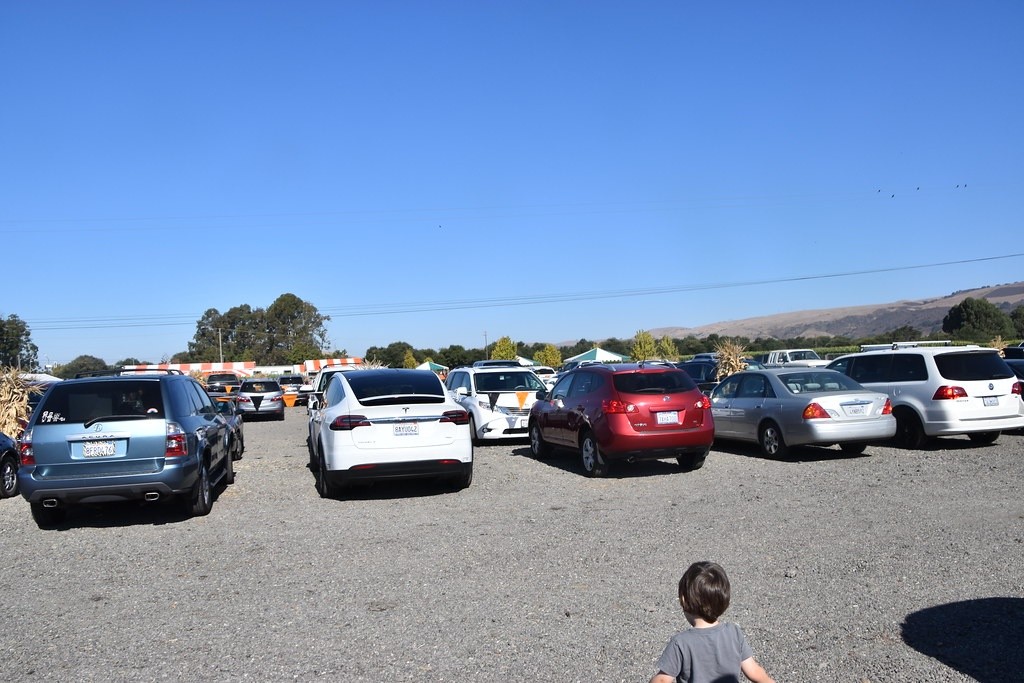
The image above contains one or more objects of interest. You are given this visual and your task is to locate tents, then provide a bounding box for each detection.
[417,361,450,378]
[563,346,632,364]
[511,354,541,367]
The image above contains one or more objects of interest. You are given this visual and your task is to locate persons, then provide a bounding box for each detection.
[649,561,775,683]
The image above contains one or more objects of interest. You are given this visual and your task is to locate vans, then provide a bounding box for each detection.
[443,365,552,446]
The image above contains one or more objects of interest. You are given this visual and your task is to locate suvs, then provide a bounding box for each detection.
[17,368,238,531]
[806,340,1024,451]
[526,361,716,479]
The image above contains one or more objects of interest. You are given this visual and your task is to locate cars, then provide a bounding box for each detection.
[205,341,860,461]
[0,431,21,499]
[706,366,898,462]
[308,367,475,498]
